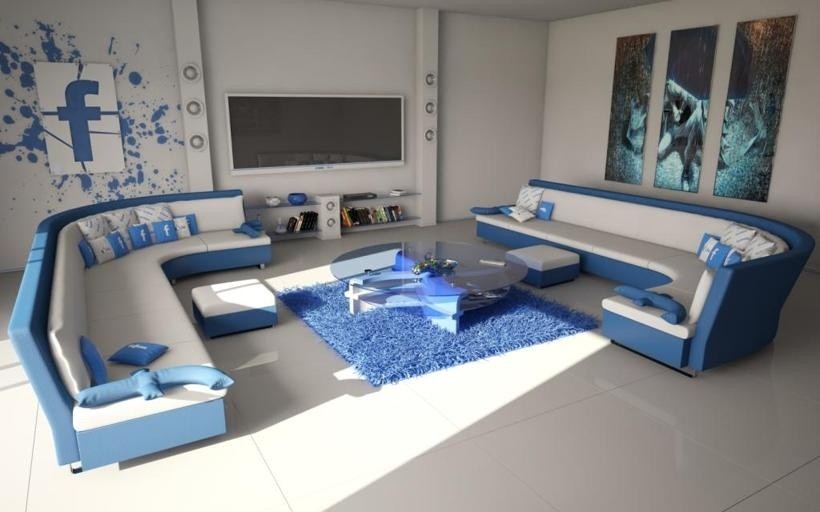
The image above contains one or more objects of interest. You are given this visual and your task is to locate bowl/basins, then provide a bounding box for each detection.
[287,192,308,206]
[264,196,281,207]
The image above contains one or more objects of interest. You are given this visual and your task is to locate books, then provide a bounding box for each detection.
[287,204,404,233]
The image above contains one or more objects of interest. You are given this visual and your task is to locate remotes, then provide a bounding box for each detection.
[479,258,507,267]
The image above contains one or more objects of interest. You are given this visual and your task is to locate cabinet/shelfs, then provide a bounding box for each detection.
[244,193,425,241]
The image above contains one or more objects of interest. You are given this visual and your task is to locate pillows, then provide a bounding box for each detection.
[231,219,263,239]
[77,333,237,407]
[695,222,777,271]
[471,186,555,224]
[76,202,202,268]
[613,283,687,325]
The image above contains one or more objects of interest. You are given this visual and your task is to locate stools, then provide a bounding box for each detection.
[504,245,582,290]
[191,278,278,339]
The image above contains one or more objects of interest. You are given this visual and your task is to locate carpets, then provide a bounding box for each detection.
[276,263,601,387]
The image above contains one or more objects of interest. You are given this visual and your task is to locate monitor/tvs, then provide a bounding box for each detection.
[224,94,405,176]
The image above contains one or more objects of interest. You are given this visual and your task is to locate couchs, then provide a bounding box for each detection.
[475,177,815,379]
[7,189,273,474]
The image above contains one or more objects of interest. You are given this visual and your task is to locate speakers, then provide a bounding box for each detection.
[424,71,437,144]
[180,63,208,152]
[316,195,341,241]
